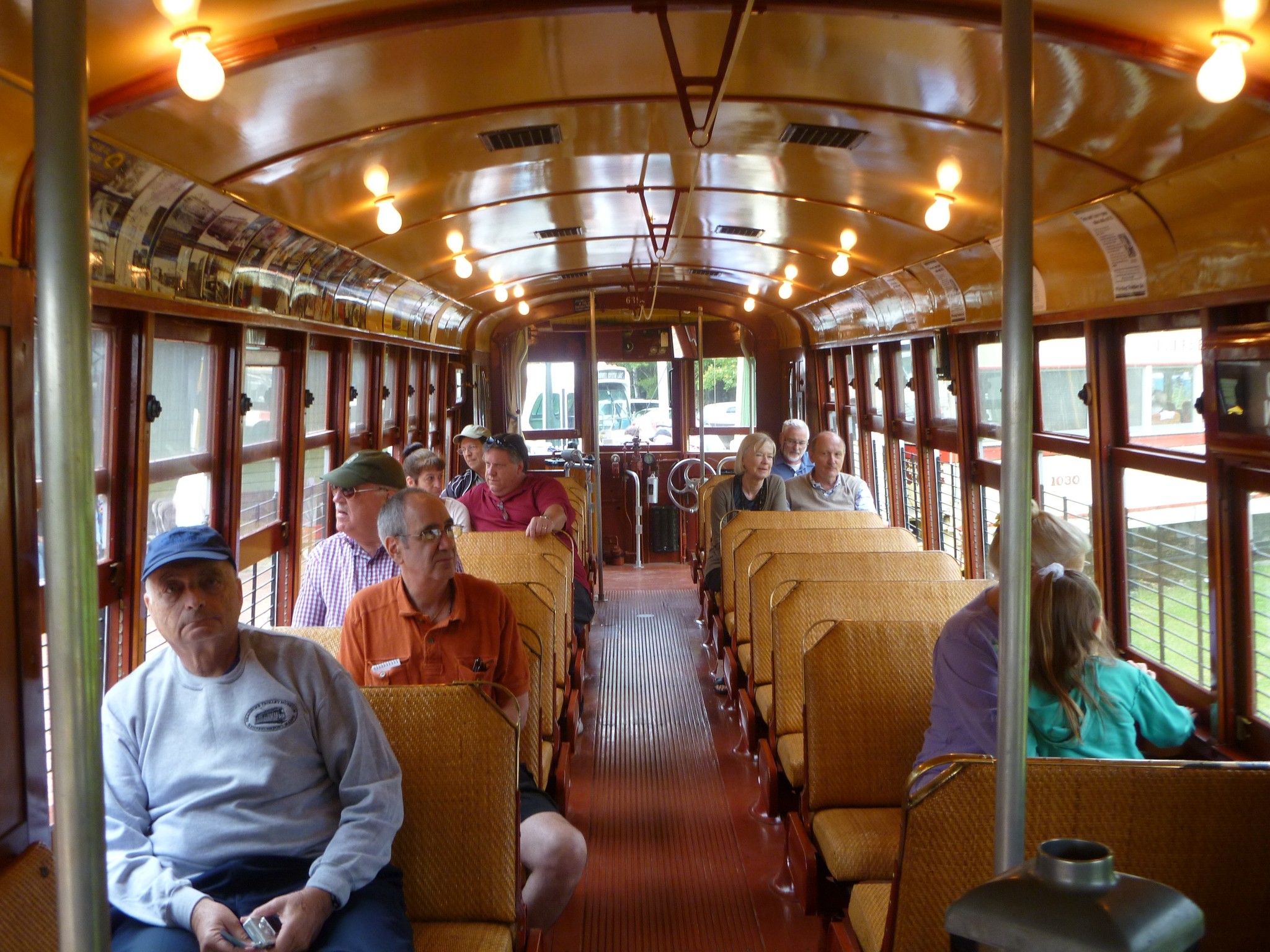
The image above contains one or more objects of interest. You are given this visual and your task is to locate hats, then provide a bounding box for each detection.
[141,525,236,582]
[318,450,407,489]
[453,424,491,444]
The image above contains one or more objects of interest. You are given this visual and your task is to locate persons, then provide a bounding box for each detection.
[440,424,493,500]
[338,489,588,931]
[455,433,595,640]
[103,526,415,952]
[290,448,465,628]
[907,498,1198,794]
[783,431,877,513]
[770,418,815,481]
[400,442,471,534]
[703,432,790,695]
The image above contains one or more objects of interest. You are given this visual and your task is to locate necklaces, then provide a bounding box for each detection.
[739,477,763,511]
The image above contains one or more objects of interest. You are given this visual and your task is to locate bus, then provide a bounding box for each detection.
[833,328,1270,531]
[519,361,631,456]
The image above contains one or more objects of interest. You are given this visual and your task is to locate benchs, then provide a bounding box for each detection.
[691,476,1270,952]
[262,477,597,952]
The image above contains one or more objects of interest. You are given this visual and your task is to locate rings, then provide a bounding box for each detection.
[542,526,547,529]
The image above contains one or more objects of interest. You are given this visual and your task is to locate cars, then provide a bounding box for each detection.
[598,398,738,451]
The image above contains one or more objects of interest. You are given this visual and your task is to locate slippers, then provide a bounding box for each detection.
[714,677,728,694]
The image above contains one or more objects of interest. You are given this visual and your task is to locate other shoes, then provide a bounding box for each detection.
[577,717,583,734]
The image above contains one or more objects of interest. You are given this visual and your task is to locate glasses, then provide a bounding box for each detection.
[457,443,485,455]
[783,434,808,448]
[393,524,464,542]
[496,498,509,520]
[330,483,389,499]
[486,436,522,455]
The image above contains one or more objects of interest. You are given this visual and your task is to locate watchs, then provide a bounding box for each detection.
[540,515,549,519]
